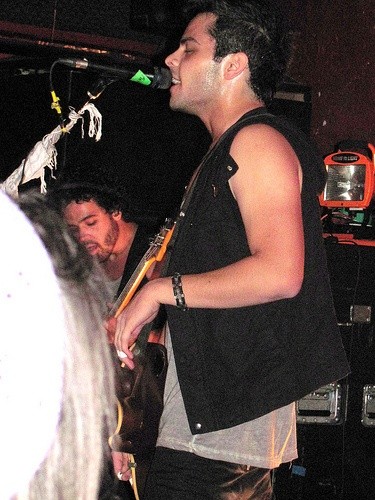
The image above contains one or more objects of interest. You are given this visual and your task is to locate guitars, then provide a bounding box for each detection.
[104,217,177,323]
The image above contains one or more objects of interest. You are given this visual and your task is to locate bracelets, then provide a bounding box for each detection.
[170,269,189,313]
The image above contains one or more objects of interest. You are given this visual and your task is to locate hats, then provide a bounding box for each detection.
[52,164,121,194]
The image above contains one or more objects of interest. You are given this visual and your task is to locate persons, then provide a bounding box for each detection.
[113,0,349,499]
[1,187,115,500]
[51,165,168,343]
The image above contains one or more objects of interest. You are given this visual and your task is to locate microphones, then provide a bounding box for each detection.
[56,57,172,90]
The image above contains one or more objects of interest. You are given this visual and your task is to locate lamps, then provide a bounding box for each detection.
[318,139,375,240]
